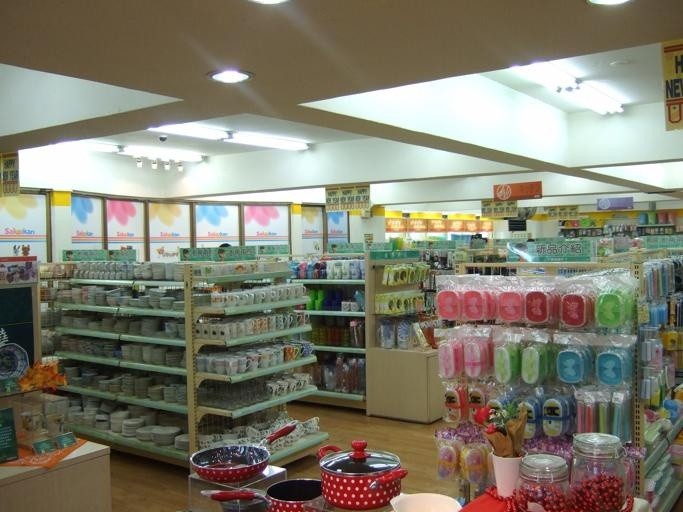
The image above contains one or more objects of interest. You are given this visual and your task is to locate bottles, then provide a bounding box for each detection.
[334,301,365,394]
[642,259,676,326]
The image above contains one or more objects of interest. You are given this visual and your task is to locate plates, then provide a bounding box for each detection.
[390,492,463,512]
[122,417,189,452]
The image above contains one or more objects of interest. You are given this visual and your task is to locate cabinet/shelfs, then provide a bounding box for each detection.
[1,433,111,512]
[41,248,682,512]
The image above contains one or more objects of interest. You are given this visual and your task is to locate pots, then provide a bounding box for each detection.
[189,422,407,512]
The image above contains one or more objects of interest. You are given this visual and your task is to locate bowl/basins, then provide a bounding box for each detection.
[62,285,186,406]
[110,411,130,434]
[0,343,30,381]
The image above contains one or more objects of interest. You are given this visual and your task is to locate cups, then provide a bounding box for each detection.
[73,260,184,282]
[492,451,527,499]
[21,411,64,436]
[374,319,418,351]
[197,282,321,456]
